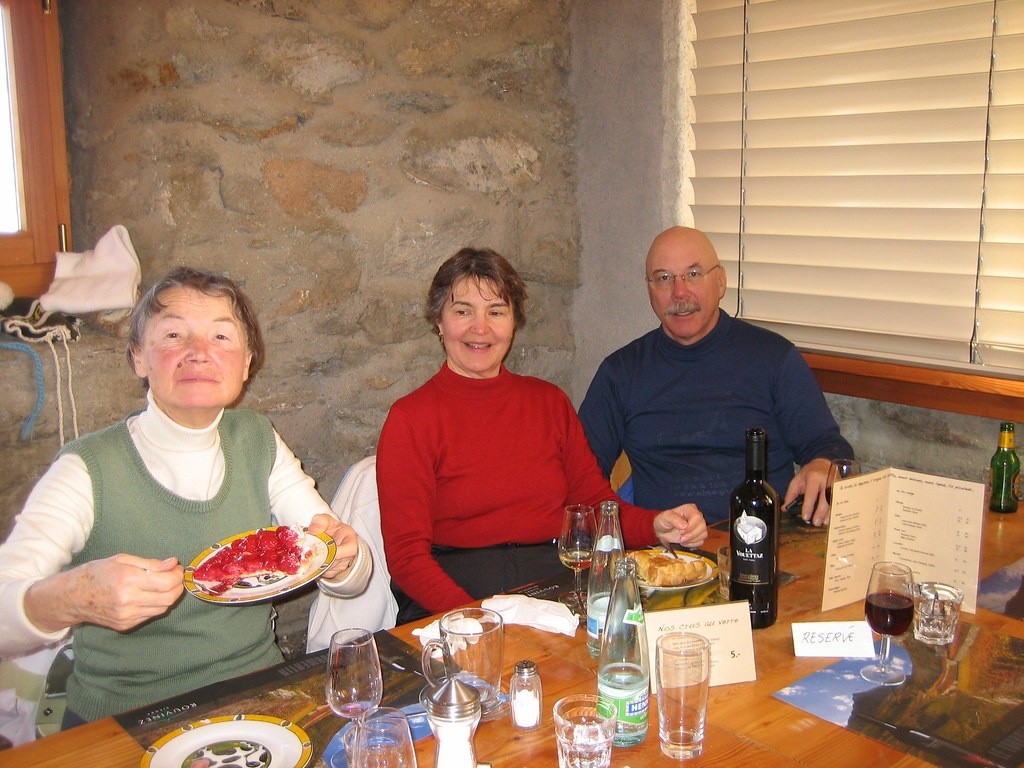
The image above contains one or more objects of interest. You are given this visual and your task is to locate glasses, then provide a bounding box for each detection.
[645,265,724,291]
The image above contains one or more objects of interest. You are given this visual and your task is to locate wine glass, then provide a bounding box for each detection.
[859,562,914,685]
[325,627,383,767]
[557,504,597,608]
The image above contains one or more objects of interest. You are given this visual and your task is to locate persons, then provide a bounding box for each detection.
[376,247,709,626]
[0,268,374,737]
[577,226,854,525]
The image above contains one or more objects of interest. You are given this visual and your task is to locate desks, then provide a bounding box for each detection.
[0,489,1024,768]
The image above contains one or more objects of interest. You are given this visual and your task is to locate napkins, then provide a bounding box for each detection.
[481,593,580,638]
[412,611,483,658]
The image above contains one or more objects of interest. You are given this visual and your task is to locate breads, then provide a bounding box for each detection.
[628,549,708,585]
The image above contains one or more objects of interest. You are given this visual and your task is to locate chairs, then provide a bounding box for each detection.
[301,454,399,654]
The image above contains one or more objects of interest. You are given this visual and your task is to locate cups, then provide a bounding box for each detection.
[716,546,730,600]
[824,459,863,508]
[654,632,711,759]
[553,694,617,768]
[913,581,964,645]
[343,707,418,768]
[420,608,503,704]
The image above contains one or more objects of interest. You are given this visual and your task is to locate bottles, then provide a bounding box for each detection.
[585,502,625,660]
[426,678,482,768]
[989,422,1019,515]
[728,427,779,629]
[597,557,649,746]
[509,660,542,729]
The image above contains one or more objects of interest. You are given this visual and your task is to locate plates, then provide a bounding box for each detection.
[182,527,337,603]
[627,549,719,591]
[418,673,511,721]
[139,713,314,768]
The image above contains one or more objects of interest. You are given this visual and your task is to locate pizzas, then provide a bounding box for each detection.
[192,524,310,585]
